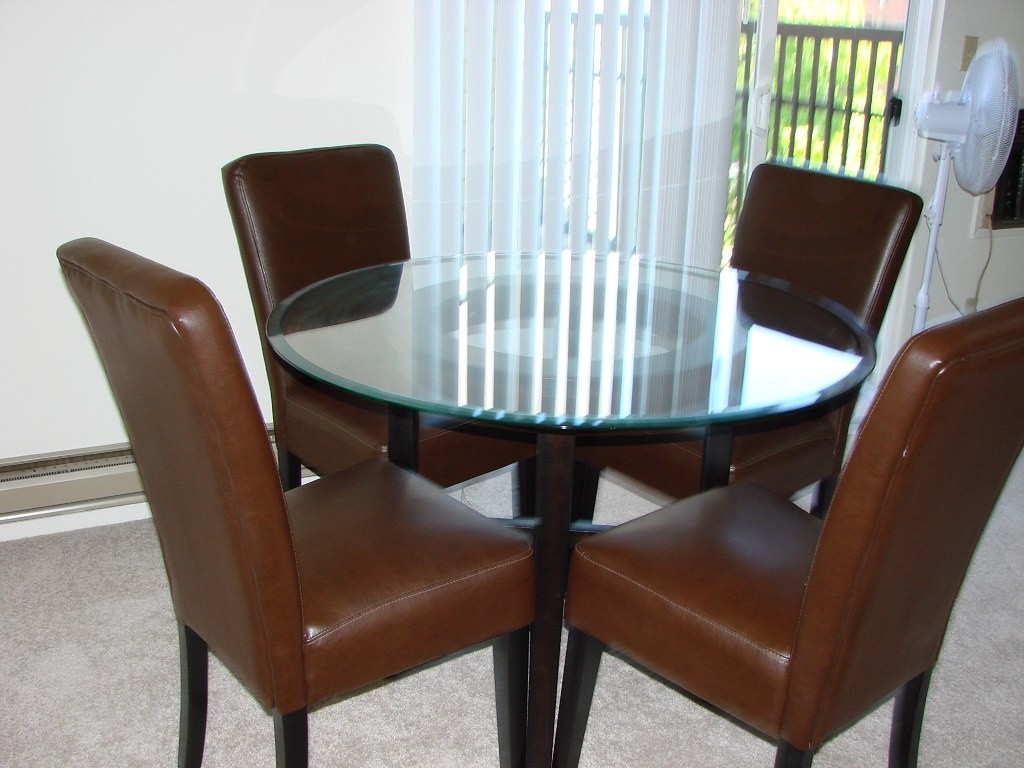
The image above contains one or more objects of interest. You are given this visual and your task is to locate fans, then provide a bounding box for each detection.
[833,38,1020,434]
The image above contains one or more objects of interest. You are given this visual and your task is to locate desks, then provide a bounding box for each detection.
[267,254,879,768]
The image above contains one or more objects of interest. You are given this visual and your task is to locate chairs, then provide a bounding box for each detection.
[57,144,1024,768]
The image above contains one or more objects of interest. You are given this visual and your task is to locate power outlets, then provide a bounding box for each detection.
[965,298,977,315]
